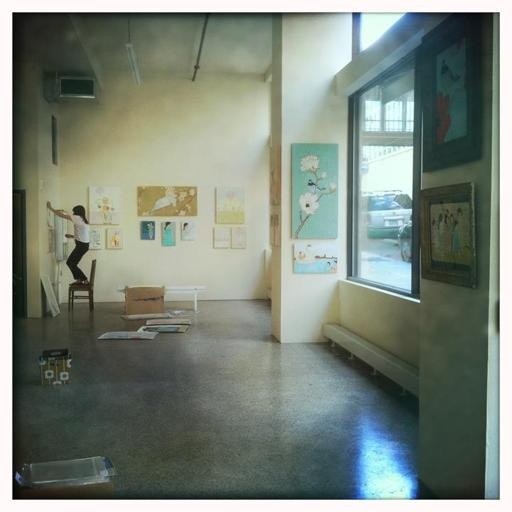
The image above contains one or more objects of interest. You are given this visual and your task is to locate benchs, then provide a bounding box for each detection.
[117,285,209,312]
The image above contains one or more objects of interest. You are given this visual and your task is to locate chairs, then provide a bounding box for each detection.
[68,259,97,312]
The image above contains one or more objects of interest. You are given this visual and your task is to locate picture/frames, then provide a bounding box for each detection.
[416,13,482,291]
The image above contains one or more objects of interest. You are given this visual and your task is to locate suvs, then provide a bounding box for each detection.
[358,188,415,241]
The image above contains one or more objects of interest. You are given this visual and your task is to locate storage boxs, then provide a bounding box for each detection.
[123,284,165,315]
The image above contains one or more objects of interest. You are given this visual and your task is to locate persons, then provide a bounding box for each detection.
[47,201,90,286]
[431,208,471,258]
